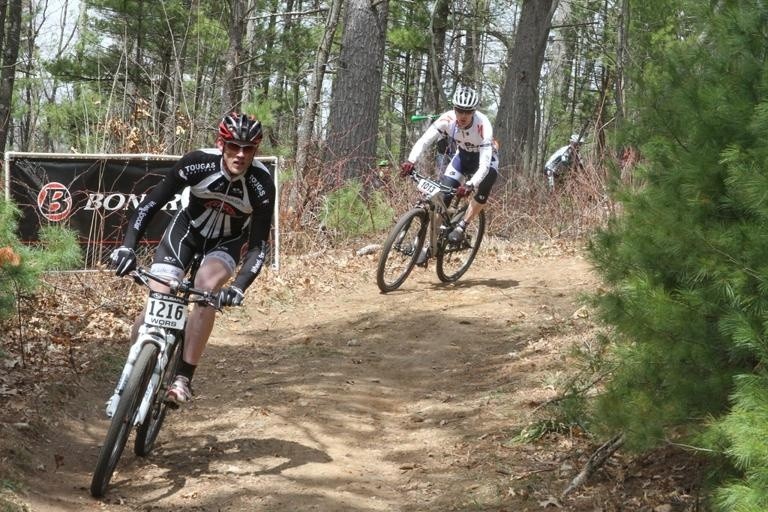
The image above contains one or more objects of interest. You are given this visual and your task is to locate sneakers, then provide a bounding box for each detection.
[414,249,433,265]
[447,225,467,244]
[162,375,195,405]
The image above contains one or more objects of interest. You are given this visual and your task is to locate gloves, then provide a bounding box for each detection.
[401,160,415,176]
[110,246,136,277]
[453,183,475,199]
[213,285,243,312]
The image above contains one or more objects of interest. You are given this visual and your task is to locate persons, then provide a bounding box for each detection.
[397,84,501,267]
[539,131,584,196]
[102,109,277,420]
[435,135,451,170]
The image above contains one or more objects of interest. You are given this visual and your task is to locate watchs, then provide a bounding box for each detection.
[464,180,475,189]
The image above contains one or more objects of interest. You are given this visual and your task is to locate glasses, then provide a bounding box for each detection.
[454,108,475,115]
[224,142,257,156]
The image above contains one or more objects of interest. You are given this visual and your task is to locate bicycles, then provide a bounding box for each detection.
[89,250,245,500]
[376,166,486,292]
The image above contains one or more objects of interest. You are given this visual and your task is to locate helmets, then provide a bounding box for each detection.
[569,133,585,144]
[452,86,479,110]
[218,111,264,144]
